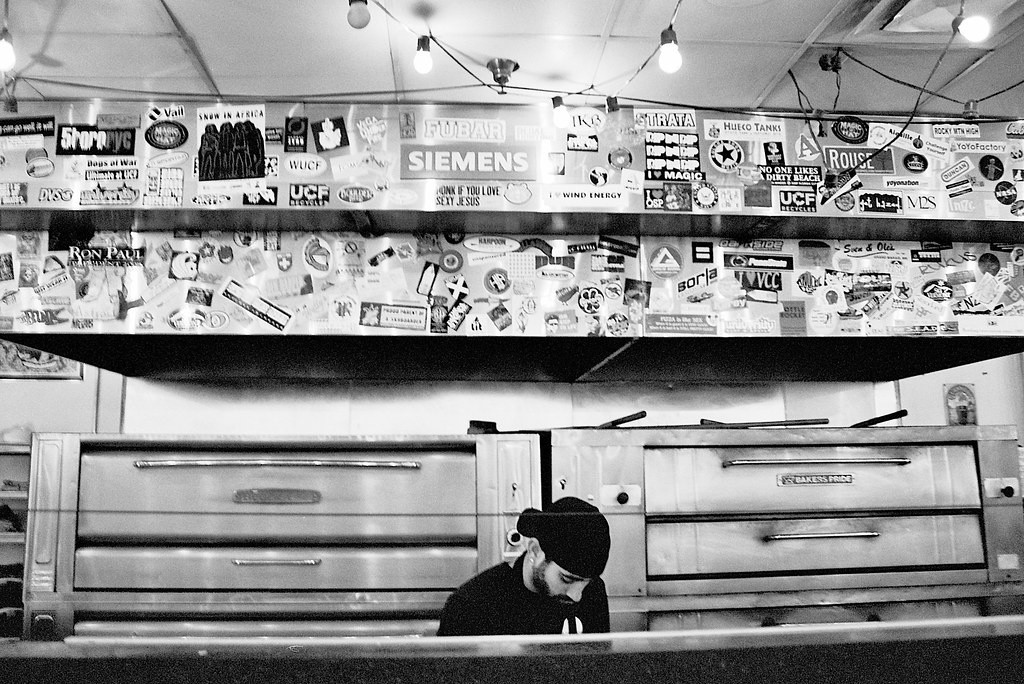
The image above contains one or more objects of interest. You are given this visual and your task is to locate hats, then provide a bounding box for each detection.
[516,497,611,577]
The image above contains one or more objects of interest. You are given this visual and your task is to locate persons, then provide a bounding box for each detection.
[432,498,611,633]
